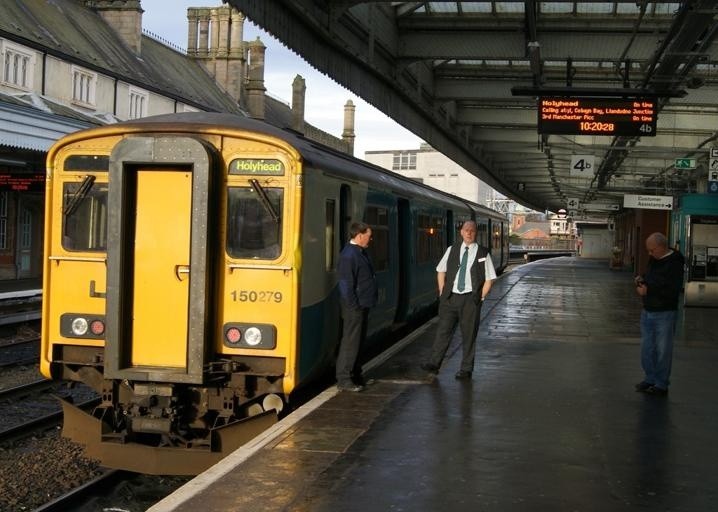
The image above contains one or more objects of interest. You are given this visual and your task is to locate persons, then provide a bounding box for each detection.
[633,230,683,398]
[421,219,497,382]
[332,220,379,393]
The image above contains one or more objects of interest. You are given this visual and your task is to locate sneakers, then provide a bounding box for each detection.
[418,362,439,375]
[454,371,472,378]
[634,381,670,402]
[338,375,375,392]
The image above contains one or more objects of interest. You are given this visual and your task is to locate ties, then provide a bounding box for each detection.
[456,245,471,292]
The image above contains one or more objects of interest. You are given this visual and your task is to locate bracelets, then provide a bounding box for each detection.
[480,297,486,301]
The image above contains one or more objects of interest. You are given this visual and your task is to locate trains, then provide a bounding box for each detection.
[35,110,514,479]
